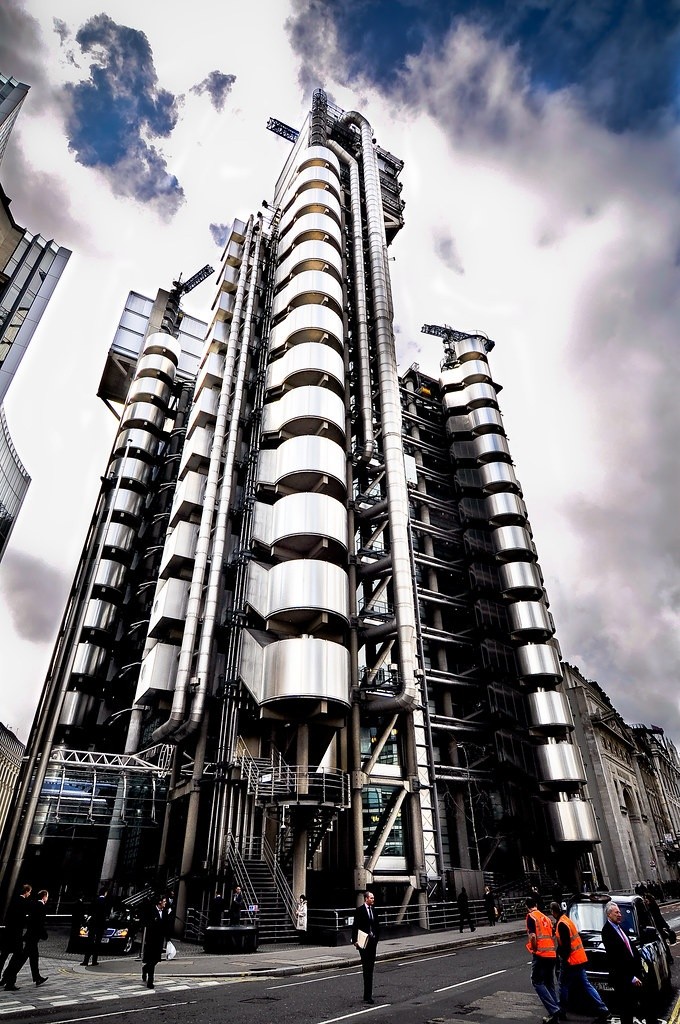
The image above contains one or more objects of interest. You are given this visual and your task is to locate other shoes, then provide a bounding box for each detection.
[36,977,49,986]
[460,930,463,933]
[543,1013,560,1024]
[4,985,20,991]
[142,967,147,981]
[80,963,88,966]
[92,963,99,966]
[147,984,154,989]
[670,958,673,965]
[592,1011,613,1024]
[363,996,374,1004]
[472,928,477,932]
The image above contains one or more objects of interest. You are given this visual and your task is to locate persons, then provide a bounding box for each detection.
[0,884,49,991]
[581,881,590,892]
[484,887,504,927]
[635,879,680,902]
[643,893,676,964]
[458,887,476,933]
[552,882,563,906]
[207,891,225,926]
[142,891,177,989]
[532,887,546,910]
[229,886,243,926]
[350,891,379,1003]
[593,880,609,891]
[601,902,658,1024]
[549,902,612,1024]
[296,894,307,945]
[526,897,564,1024]
[71,887,108,966]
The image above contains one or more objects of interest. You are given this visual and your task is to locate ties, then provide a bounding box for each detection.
[368,907,374,936]
[618,926,631,951]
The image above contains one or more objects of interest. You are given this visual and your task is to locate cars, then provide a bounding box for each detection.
[561,893,673,1012]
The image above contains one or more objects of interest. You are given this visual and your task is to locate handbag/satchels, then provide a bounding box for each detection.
[165,941,176,960]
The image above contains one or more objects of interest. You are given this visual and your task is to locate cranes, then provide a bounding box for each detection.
[266,117,302,144]
[171,264,215,299]
[421,323,497,370]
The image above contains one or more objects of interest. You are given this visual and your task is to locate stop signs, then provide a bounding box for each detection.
[649,861,655,867]
